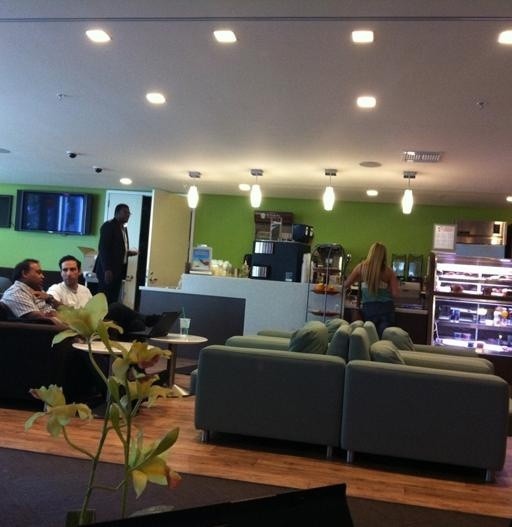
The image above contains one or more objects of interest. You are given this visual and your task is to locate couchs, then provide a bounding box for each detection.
[0,277,144,417]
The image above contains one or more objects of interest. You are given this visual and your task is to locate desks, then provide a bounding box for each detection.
[71,340,162,419]
[145,328,213,401]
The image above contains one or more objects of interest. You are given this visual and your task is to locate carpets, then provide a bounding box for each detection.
[0,446,512,527]
[173,363,199,378]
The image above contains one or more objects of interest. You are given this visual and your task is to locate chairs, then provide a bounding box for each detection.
[193,315,346,469]
[349,315,497,376]
[73,480,356,527]
[342,322,510,489]
[256,315,345,338]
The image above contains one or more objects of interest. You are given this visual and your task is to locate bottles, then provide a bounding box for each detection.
[493,307,511,324]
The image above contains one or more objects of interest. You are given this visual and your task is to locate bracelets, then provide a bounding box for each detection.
[46,294,54,304]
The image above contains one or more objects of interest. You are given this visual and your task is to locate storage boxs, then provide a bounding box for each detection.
[77,246,98,271]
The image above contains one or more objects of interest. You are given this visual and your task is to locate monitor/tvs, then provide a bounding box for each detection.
[14,190,93,235]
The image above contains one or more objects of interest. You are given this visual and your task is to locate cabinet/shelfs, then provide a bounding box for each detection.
[424,247,512,379]
[304,241,348,326]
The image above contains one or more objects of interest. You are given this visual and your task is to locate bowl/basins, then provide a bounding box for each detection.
[291,221,315,242]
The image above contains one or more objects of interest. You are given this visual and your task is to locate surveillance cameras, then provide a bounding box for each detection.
[67,152,77,160]
[93,166,104,175]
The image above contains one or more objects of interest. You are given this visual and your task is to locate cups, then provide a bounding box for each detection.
[463,332,471,340]
[210,257,240,276]
[285,271,293,281]
[179,316,191,337]
[455,332,462,340]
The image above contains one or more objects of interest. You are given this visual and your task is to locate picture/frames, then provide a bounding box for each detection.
[432,221,459,252]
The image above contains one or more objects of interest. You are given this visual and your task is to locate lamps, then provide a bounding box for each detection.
[246,168,265,208]
[397,169,417,216]
[185,170,203,209]
[320,167,338,211]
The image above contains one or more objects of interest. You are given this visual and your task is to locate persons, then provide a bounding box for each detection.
[0,259,66,320]
[46,255,93,309]
[92,204,137,302]
[345,242,399,340]
